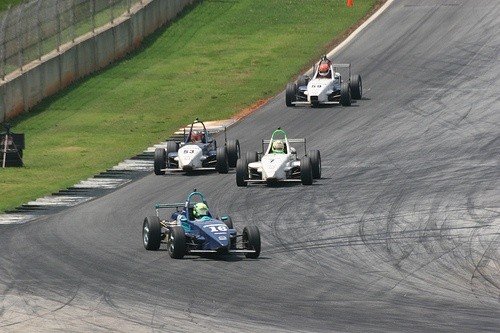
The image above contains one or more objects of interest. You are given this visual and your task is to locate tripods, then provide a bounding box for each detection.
[0,133,25,169]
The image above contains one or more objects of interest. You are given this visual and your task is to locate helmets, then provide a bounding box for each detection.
[272,141,284,153]
[193,203,209,217]
[319,64,331,77]
[191,131,200,140]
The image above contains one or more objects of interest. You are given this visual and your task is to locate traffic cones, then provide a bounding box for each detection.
[347,0,353,7]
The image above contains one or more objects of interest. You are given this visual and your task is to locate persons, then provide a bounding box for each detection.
[319,64,330,78]
[193,202,208,220]
[272,140,284,154]
[191,131,200,143]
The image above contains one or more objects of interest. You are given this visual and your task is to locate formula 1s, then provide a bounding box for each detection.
[286,53,362,107]
[142,188,261,259]
[235,127,322,187]
[153,118,241,175]
